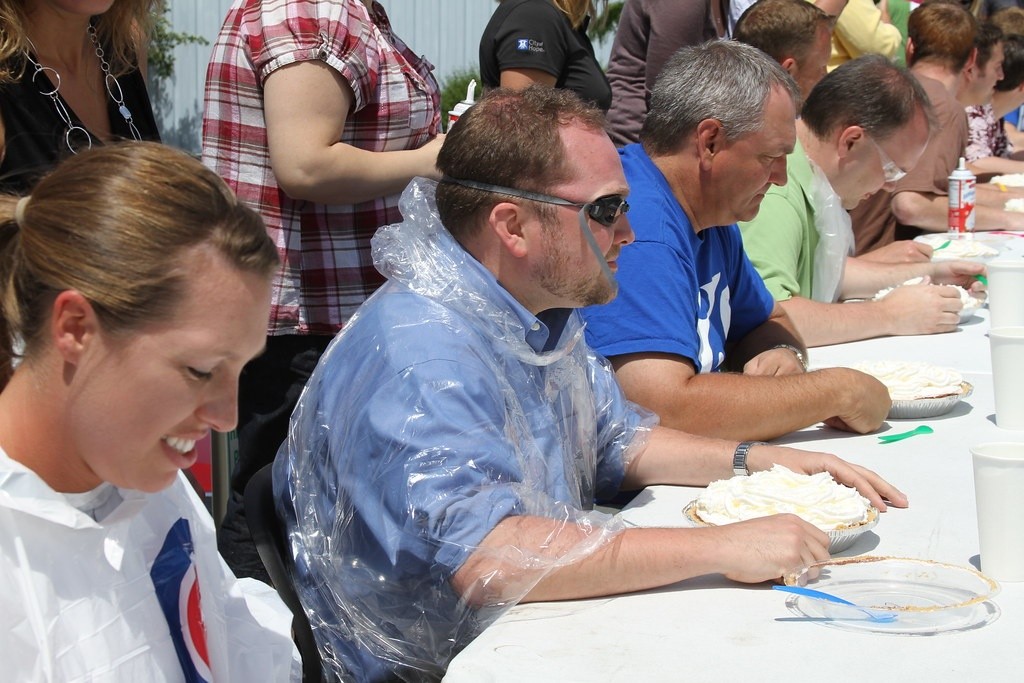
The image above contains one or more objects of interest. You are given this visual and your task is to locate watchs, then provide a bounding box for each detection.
[734,440,767,476]
[769,343,808,370]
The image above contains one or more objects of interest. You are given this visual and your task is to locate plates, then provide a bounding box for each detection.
[806,356,972,417]
[682,497,880,556]
[958,301,981,325]
[783,556,996,634]
[913,233,1024,264]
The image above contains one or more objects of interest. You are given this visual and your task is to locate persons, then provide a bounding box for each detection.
[604,0,1024,258]
[473,0,617,121]
[202,0,451,586]
[0,143,304,683]
[734,50,988,349]
[272,81,908,683]
[0,0,205,397]
[573,35,894,443]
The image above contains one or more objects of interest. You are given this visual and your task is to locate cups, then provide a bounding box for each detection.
[983,259,1024,332]
[969,439,1024,582]
[986,326,1024,430]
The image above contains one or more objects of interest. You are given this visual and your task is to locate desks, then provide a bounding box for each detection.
[450,168,1024,681]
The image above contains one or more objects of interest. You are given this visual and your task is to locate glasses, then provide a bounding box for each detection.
[589,193,632,227]
[863,130,908,183]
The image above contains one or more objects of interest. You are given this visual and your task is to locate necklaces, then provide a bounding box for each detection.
[15,17,142,155]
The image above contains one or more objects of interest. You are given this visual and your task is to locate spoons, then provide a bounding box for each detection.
[772,584,897,624]
[877,425,933,443]
[932,239,951,251]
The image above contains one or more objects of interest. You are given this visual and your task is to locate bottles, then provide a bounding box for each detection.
[946,157,975,233]
[447,79,478,136]
